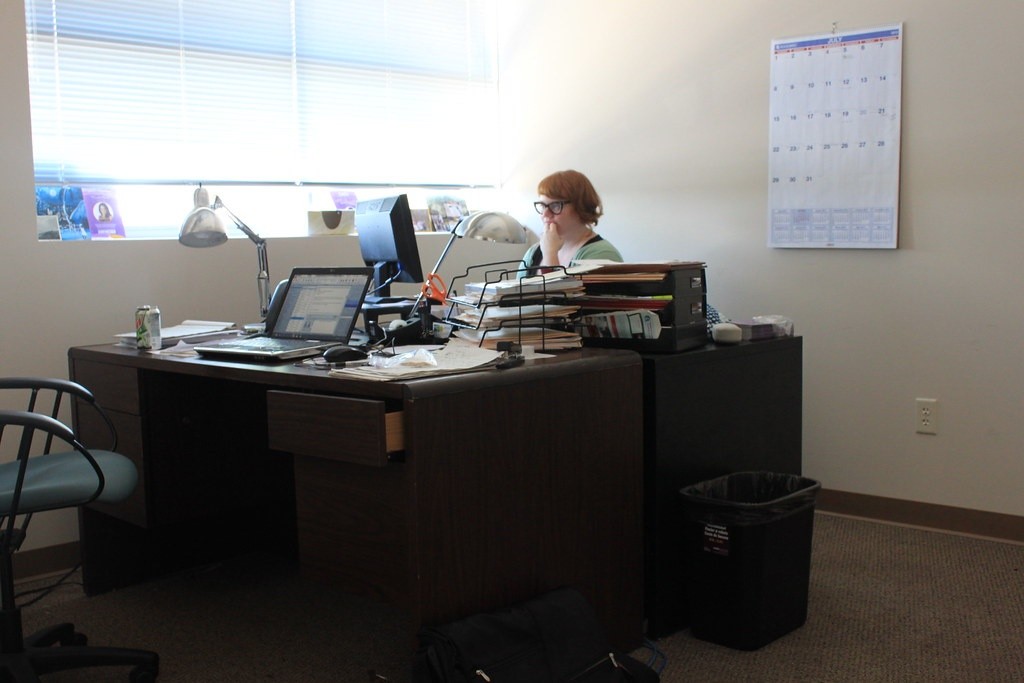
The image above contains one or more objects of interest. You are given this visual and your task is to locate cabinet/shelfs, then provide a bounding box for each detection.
[574,259,707,356]
[445,260,583,355]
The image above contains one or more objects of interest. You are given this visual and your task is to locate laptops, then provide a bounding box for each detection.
[193,267,375,361]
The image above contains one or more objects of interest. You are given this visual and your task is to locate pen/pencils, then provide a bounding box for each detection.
[314,361,370,371]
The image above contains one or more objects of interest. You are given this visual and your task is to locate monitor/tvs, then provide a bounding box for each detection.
[355,194,424,304]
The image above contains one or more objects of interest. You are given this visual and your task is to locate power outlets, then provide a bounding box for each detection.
[915,398,938,435]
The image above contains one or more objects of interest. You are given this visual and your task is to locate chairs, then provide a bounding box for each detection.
[0,376,163,683]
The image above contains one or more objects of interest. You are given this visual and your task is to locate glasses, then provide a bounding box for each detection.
[534,200,573,214]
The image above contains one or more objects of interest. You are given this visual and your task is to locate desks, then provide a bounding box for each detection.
[68,321,803,683]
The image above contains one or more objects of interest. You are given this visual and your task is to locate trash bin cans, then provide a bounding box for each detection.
[680,472,822,652]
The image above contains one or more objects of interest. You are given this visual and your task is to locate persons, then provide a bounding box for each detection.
[517,170,624,277]
[98,204,112,222]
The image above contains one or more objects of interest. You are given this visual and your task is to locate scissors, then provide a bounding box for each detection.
[422,272,448,305]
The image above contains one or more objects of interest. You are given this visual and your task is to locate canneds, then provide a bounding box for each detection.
[135,304,162,351]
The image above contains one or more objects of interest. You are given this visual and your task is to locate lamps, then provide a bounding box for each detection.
[408,211,527,318]
[178,187,273,323]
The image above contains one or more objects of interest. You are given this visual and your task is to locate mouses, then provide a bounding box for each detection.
[323,346,369,363]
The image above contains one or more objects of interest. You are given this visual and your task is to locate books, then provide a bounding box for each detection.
[444,278,586,349]
[112,319,237,350]
